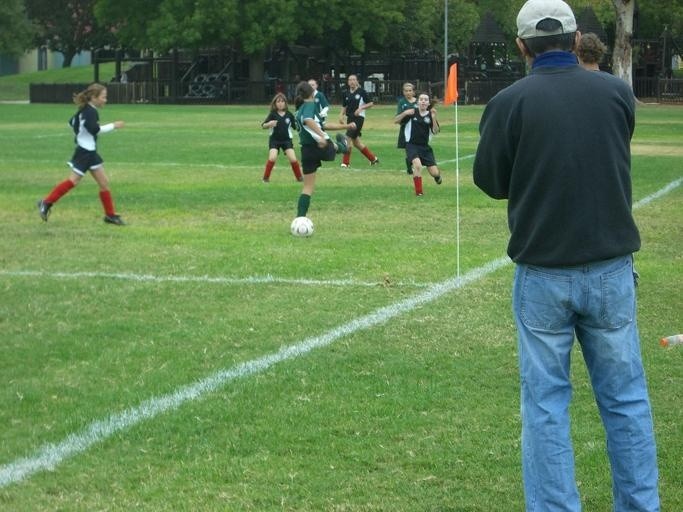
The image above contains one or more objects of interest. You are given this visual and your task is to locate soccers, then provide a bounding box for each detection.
[289,216,313,236]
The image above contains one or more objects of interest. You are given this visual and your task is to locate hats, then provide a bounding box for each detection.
[515,0,578,40]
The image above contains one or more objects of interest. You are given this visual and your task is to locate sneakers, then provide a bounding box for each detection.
[435,176,443,185]
[37,200,52,221]
[341,163,350,168]
[104,213,123,225]
[262,176,269,183]
[336,133,349,154]
[416,193,424,197]
[371,156,379,166]
[296,176,304,182]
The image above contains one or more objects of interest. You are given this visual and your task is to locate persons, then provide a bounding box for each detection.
[339,74,380,168]
[576,32,606,72]
[307,79,328,132]
[295,81,357,217]
[397,81,418,148]
[261,92,303,182]
[394,91,442,196]
[472,0,661,511]
[38,82,127,226]
